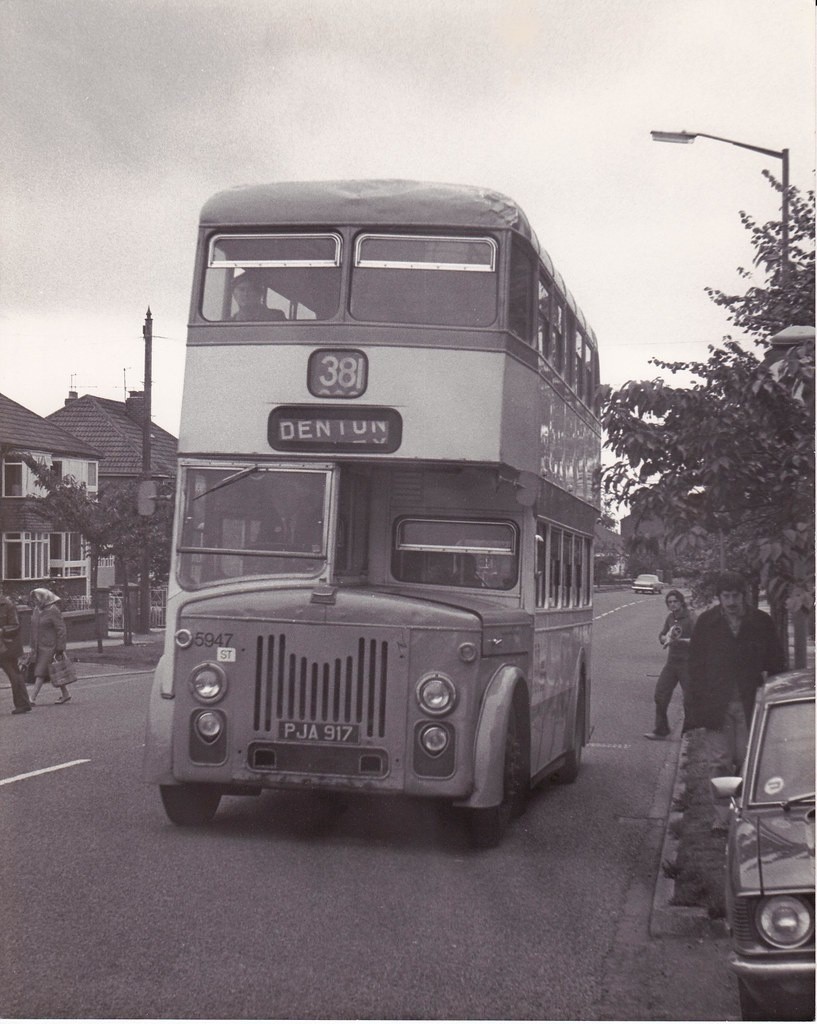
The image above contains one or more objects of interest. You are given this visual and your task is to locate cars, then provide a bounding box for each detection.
[710,664,817,1020]
[631,574,665,595]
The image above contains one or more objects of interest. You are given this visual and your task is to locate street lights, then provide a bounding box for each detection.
[649,127,805,663]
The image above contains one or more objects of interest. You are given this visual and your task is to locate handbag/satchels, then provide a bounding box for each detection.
[48,652,78,687]
[20,663,50,683]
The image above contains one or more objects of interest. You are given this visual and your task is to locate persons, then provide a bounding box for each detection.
[227,271,286,322]
[1,580,32,715]
[27,587,71,707]
[445,553,483,589]
[687,571,786,797]
[242,478,321,555]
[643,591,699,740]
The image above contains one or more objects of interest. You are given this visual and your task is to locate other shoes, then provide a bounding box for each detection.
[11,703,32,714]
[711,819,729,838]
[643,732,673,741]
[28,698,36,706]
[55,693,72,704]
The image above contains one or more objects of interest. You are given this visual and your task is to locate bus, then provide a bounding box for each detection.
[131,181,607,842]
